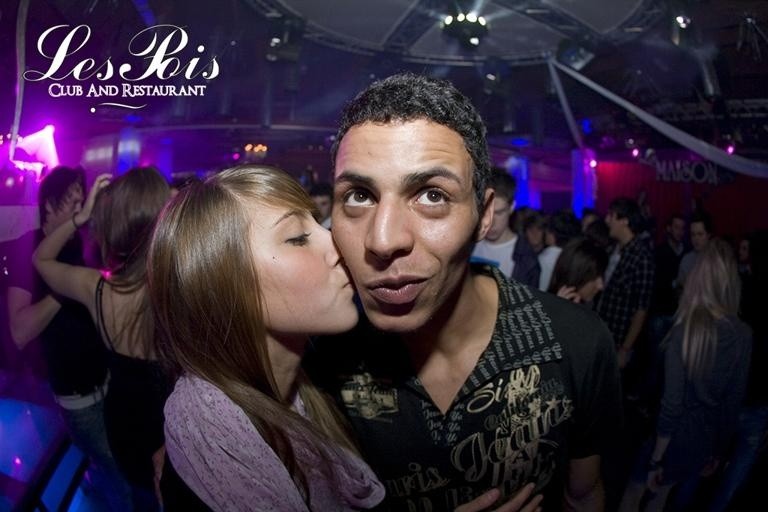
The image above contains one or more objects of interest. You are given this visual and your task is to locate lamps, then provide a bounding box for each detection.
[437,14,488,49]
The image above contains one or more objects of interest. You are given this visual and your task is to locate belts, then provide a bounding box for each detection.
[52,382,108,411]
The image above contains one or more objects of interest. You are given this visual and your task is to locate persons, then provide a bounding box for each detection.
[296,69,633,509]
[0,159,767,512]
